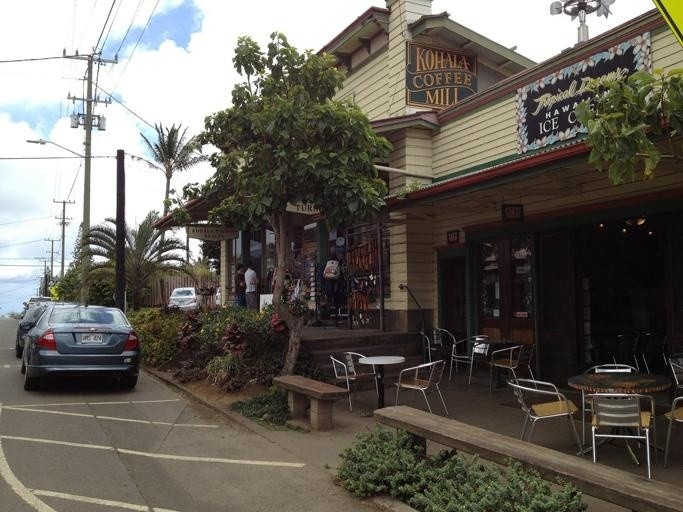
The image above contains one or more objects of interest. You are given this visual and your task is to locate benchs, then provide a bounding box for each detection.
[273,373,349,436]
[372,403,682,512]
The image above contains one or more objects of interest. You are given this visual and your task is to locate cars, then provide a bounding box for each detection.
[15,297,74,356]
[166,286,202,313]
[19,304,140,392]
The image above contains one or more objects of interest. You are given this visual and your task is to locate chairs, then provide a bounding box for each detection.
[489,344,539,392]
[506,378,587,460]
[584,393,656,480]
[420,327,458,374]
[611,326,682,397]
[393,358,450,418]
[583,363,638,375]
[663,396,682,466]
[449,335,491,384]
[329,351,381,411]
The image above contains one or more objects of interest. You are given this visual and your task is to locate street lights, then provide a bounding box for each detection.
[26,137,91,306]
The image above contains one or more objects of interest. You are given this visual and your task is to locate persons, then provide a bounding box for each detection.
[245,259,260,310]
[234,263,246,307]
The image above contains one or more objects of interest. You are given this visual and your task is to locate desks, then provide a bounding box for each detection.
[568,373,673,465]
[357,356,404,409]
